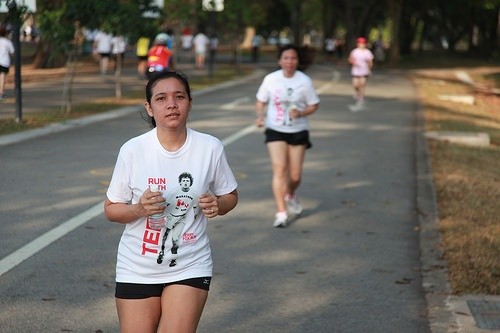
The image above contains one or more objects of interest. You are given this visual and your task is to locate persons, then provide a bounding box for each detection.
[134,29,177,87]
[180,26,219,70]
[84,24,126,78]
[251,33,261,63]
[104,70,240,333]
[324,33,347,62]
[0,24,15,98]
[347,37,374,106]
[256,43,320,227]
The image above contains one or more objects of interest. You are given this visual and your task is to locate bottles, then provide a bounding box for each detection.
[149,184,166,229]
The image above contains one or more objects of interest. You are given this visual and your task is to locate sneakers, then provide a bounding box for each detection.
[273,211,287,227]
[285,195,302,214]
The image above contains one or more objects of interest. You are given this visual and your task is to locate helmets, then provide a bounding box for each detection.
[155,34,168,44]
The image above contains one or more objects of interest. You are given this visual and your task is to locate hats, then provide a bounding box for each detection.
[357,37,366,43]
